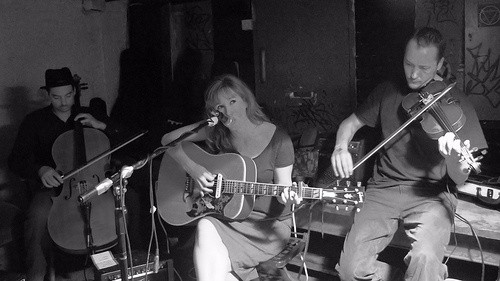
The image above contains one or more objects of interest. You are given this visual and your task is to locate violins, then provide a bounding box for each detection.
[403,80,487,175]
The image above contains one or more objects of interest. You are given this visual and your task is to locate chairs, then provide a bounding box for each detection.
[256,150,320,281]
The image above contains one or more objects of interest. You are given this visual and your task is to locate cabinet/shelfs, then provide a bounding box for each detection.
[291,190,500,280]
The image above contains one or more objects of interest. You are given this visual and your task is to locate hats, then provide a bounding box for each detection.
[41,66,79,90]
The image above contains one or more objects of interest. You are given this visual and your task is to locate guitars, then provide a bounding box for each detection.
[156,142,366,225]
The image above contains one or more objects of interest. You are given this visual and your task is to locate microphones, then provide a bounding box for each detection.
[210,110,234,126]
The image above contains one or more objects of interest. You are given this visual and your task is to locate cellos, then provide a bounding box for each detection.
[48,71,127,254]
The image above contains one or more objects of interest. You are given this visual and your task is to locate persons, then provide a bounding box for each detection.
[160,75,294,281]
[10,66,124,281]
[331,26,486,281]
